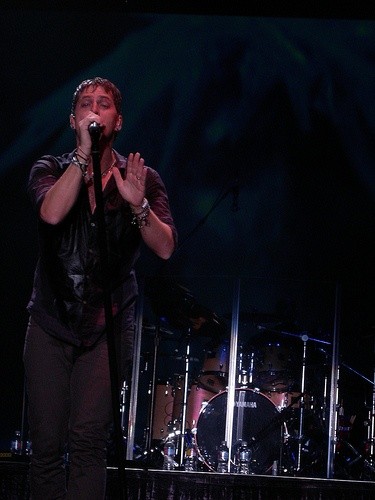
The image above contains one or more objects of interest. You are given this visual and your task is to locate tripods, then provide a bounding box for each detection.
[132,325,375,481]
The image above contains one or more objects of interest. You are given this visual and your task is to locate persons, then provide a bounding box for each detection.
[22,77,178,500]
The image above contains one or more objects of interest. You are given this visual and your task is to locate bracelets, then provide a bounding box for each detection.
[70,148,89,168]
[130,198,150,228]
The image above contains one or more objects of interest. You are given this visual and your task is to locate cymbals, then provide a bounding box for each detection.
[154,275,233,333]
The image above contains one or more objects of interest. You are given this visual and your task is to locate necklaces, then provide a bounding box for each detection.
[98,159,117,176]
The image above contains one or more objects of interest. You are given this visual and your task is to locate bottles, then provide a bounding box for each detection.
[24,431,32,455]
[161,436,176,471]
[184,438,198,472]
[237,441,250,474]
[12,431,22,455]
[217,440,228,473]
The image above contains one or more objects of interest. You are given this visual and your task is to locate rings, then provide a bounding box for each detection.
[137,177,141,180]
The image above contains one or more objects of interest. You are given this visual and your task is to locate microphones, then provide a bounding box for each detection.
[230,168,241,211]
[187,325,193,336]
[316,345,342,362]
[88,121,100,134]
[257,325,288,336]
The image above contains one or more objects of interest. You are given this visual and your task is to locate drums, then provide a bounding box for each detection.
[268,380,325,419]
[168,370,231,441]
[143,379,186,447]
[192,384,284,473]
